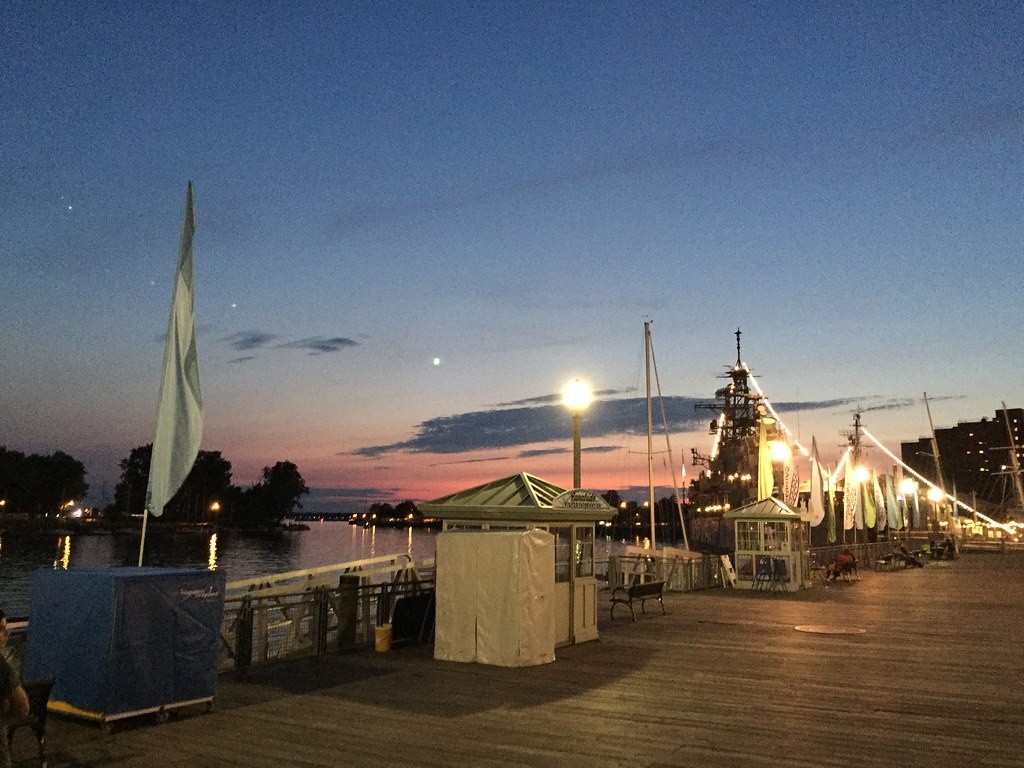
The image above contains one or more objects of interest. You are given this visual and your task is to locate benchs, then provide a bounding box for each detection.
[7,677,59,768]
[609,581,666,622]
[875,544,945,571]
[831,560,861,584]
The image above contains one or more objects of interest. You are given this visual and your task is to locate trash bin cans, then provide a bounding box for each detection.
[375,624,392,653]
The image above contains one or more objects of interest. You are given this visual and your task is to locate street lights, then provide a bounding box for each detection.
[560,378,594,576]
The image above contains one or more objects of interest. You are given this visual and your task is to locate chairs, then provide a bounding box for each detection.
[751,556,790,596]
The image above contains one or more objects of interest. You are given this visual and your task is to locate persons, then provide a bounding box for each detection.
[825,549,855,580]
[893,538,953,568]
[0,610,30,768]
